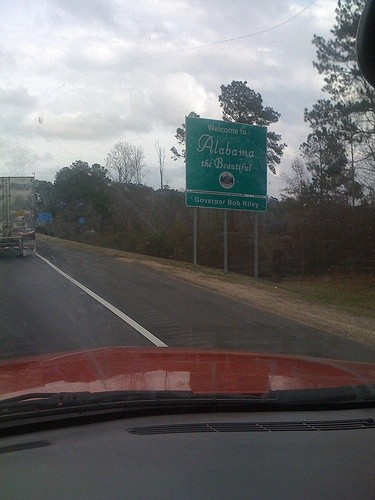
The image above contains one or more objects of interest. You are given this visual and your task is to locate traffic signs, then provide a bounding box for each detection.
[184,116,268,197]
[184,191,267,213]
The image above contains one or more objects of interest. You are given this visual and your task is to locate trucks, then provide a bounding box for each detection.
[0,175,37,258]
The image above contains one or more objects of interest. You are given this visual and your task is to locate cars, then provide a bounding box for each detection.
[0,347,375,500]
